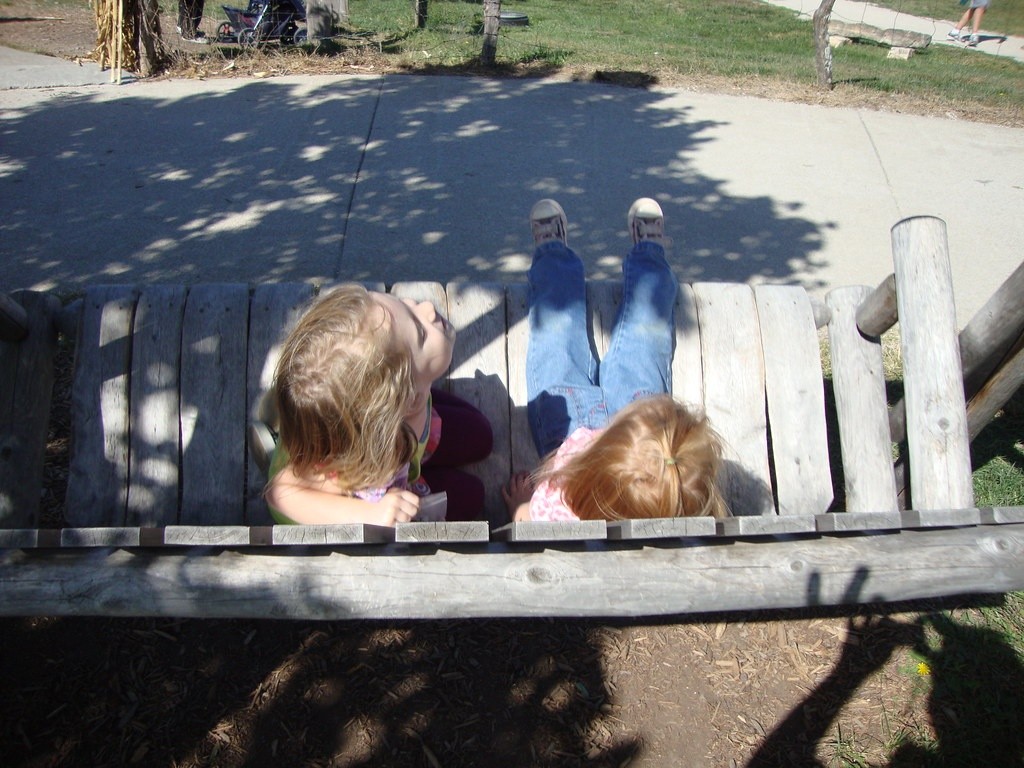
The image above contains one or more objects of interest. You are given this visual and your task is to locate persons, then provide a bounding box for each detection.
[944,0,991,47]
[499,199,733,524]
[243,282,493,529]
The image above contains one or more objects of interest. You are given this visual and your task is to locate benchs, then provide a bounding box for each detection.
[0,214,1024,621]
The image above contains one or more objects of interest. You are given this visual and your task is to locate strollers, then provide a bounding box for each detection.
[215,0,308,50]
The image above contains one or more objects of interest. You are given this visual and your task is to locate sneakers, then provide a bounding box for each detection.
[628,198,674,249]
[964,33,979,46]
[947,31,963,42]
[529,199,569,249]
